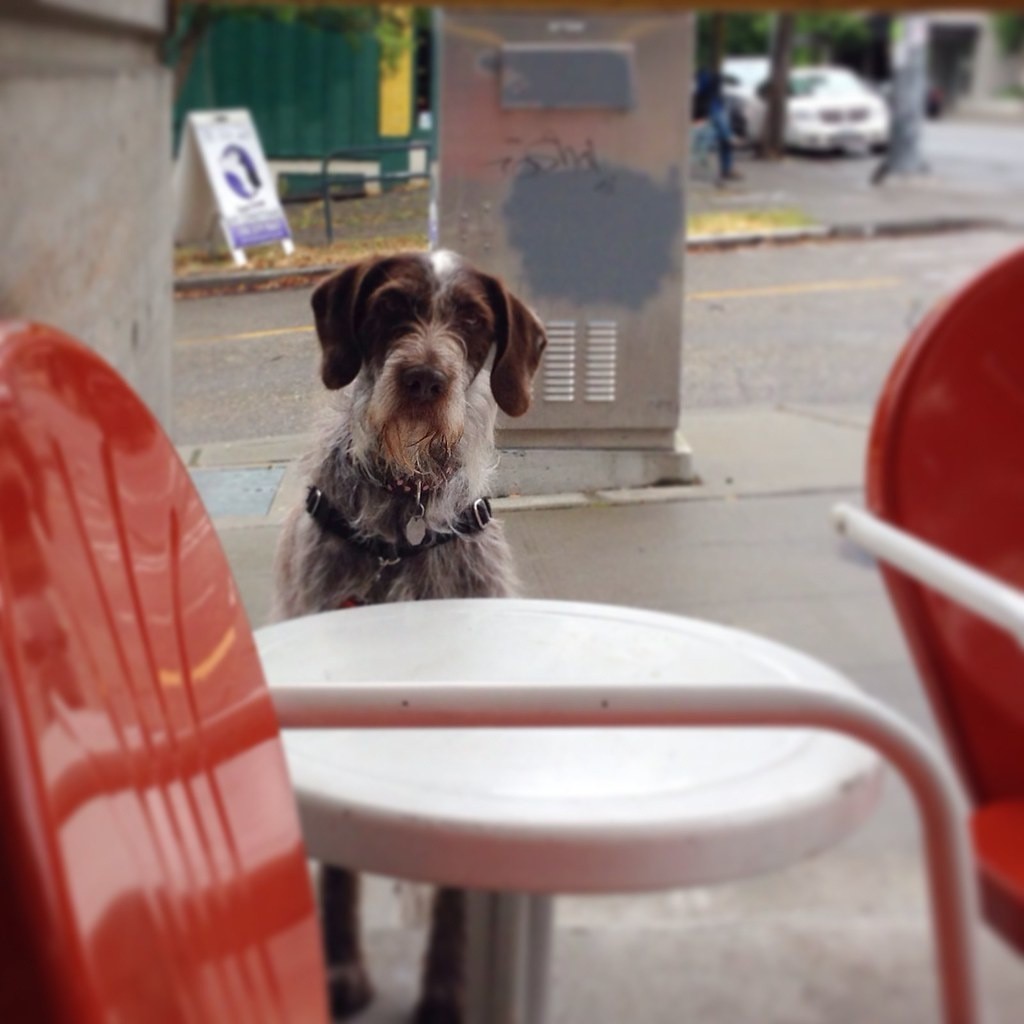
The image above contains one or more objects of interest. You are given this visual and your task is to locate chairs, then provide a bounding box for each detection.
[830,246,1024,957]
[0,316,989,1024]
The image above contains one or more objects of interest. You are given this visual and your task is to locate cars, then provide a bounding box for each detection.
[737,67,890,155]
[722,59,770,130]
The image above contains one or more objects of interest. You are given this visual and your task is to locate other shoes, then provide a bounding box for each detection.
[726,138,747,152]
[722,170,742,182]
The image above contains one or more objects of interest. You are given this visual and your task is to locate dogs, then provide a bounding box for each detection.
[275,245,548,1020]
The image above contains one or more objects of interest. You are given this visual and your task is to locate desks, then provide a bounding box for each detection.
[245,596,883,1024]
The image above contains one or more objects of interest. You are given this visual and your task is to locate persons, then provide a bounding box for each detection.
[711,109,744,179]
